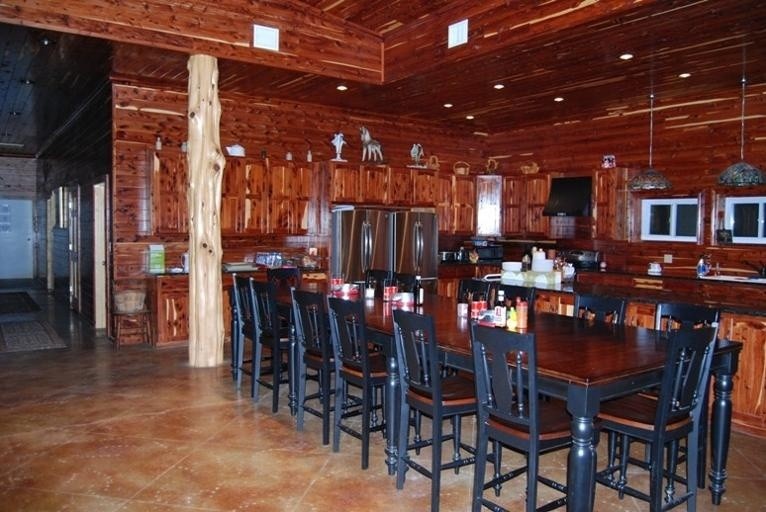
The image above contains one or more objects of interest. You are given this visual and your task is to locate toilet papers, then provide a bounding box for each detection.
[531,250,545,269]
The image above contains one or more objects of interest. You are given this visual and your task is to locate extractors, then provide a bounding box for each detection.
[542,175,594,217]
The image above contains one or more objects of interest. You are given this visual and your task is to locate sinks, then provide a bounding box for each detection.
[696,274,766,284]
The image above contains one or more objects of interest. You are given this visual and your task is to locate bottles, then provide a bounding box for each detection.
[364,277,375,298]
[523,246,546,272]
[493,288,528,330]
[412,276,425,306]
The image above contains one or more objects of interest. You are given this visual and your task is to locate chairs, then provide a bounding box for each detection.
[231,265,306,412]
[329,268,418,362]
[317,294,422,467]
[571,297,631,320]
[594,321,718,511]
[391,308,502,512]
[468,320,600,512]
[290,284,367,446]
[431,277,537,307]
[610,303,720,504]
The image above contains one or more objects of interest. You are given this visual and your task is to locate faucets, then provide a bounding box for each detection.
[741,259,766,278]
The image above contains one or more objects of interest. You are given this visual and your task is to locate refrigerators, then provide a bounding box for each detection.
[392,211,439,295]
[331,207,393,284]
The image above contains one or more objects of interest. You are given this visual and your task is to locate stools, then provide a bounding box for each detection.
[112,309,153,350]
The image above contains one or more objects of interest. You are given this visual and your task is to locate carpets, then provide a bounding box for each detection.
[0,291,43,315]
[1,317,67,352]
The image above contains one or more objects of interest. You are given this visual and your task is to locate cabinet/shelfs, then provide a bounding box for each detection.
[153,150,476,233]
[477,174,638,240]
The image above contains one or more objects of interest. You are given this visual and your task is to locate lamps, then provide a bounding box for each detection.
[717,40,763,187]
[629,36,672,191]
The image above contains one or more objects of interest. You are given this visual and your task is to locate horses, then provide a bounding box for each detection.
[359,125,383,161]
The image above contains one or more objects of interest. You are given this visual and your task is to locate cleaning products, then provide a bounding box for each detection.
[696,254,709,276]
